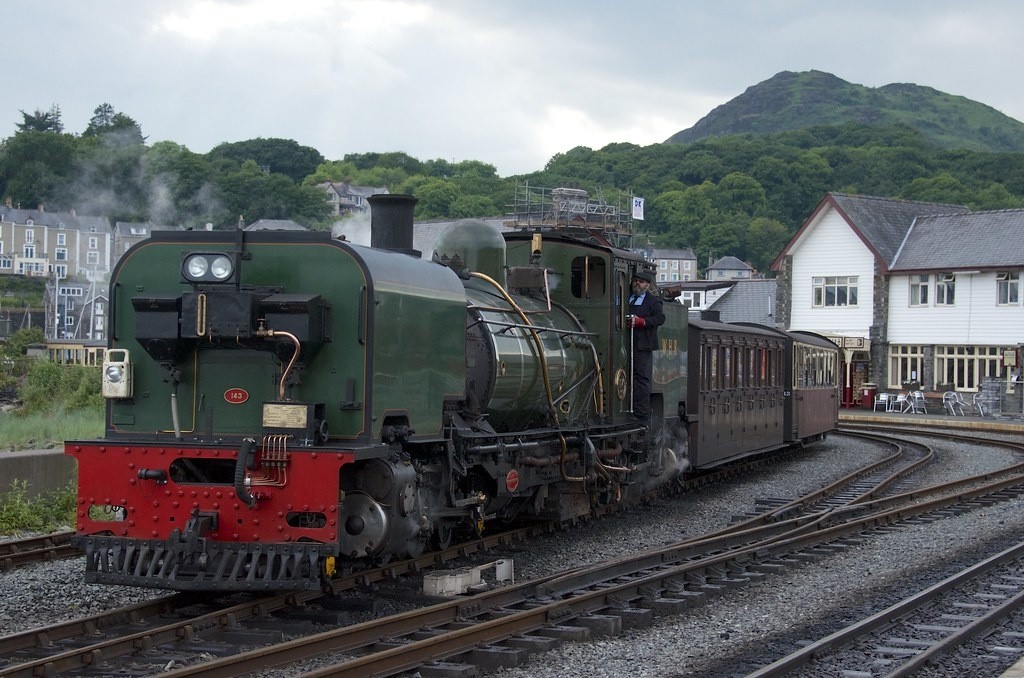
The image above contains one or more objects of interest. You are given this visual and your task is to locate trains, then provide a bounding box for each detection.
[42,178,847,592]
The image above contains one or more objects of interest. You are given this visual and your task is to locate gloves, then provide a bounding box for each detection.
[628,314,645,328]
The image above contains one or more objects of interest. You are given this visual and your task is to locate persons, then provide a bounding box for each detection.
[626,273,666,421]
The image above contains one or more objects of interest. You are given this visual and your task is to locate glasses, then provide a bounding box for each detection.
[632,277,645,283]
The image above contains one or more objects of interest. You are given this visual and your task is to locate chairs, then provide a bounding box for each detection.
[873,391,987,419]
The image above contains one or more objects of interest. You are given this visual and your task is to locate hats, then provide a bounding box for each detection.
[633,272,652,283]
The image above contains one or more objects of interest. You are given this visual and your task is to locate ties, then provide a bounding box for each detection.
[630,296,640,306]
